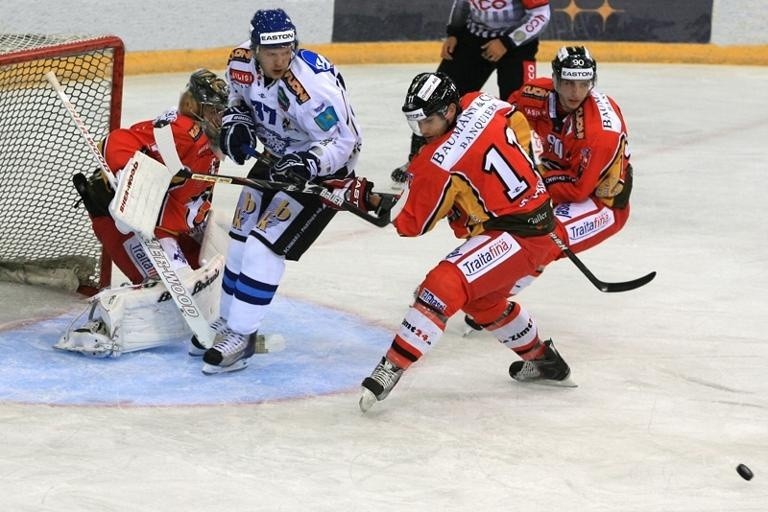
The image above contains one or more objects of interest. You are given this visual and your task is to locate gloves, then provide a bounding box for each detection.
[320,177,375,213]
[219,104,257,164]
[269,151,320,185]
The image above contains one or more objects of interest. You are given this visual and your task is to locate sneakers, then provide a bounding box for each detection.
[389,159,413,183]
[204,331,256,368]
[360,354,404,400]
[465,313,484,332]
[191,318,234,350]
[509,339,570,383]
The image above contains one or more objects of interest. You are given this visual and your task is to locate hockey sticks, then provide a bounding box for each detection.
[47,72,287,356]
[171,164,406,206]
[236,139,391,228]
[550,232,656,294]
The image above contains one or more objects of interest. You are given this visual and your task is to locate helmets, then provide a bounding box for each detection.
[250,9,297,50]
[403,73,461,138]
[179,69,230,139]
[552,45,596,95]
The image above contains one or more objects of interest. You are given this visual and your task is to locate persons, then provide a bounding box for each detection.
[358,72,578,412]
[51,69,228,360]
[459,46,634,338]
[186,9,363,376]
[389,0,551,190]
